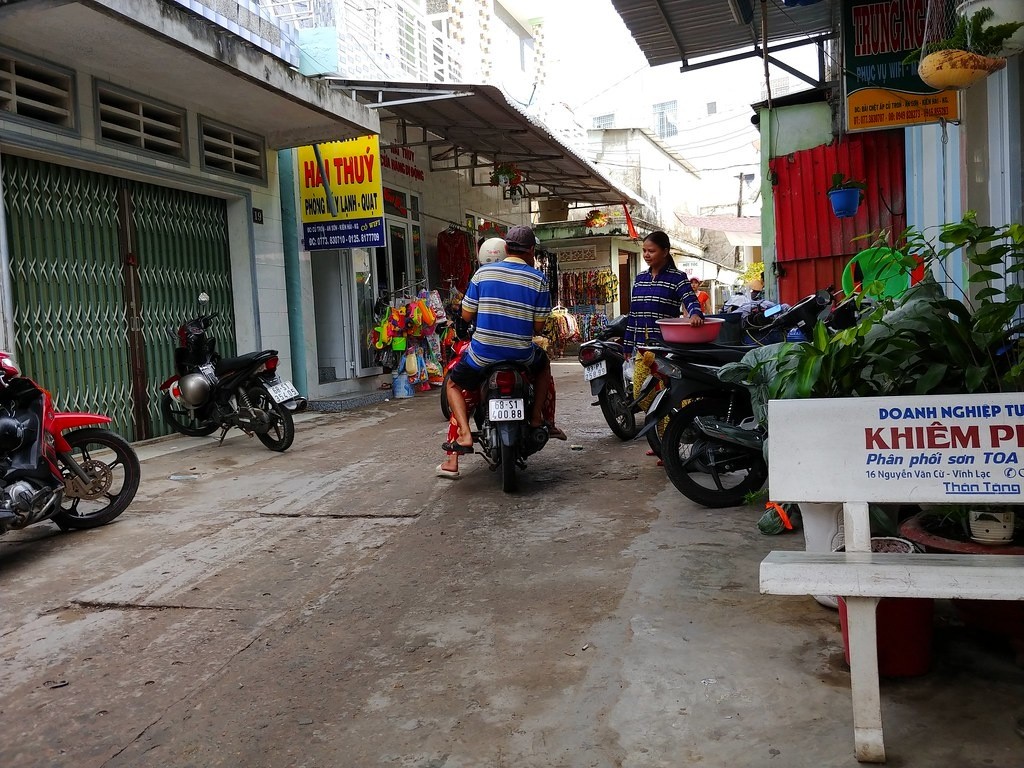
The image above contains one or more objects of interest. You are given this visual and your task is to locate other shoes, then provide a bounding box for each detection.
[657,459,663,465]
[646,449,657,455]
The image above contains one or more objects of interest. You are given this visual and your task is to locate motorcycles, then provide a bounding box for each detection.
[0,350,141,534]
[437,302,550,493]
[626,283,869,509]
[578,314,638,442]
[159,292,307,452]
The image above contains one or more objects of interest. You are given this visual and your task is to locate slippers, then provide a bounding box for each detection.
[435,462,460,477]
[547,424,568,441]
[441,440,474,453]
[529,419,552,429]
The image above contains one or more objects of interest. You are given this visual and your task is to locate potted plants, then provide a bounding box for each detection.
[712,213,1024,682]
[902,8,1024,89]
[826,173,867,216]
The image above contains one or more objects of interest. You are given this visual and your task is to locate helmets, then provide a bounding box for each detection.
[478,238,509,265]
[179,374,211,409]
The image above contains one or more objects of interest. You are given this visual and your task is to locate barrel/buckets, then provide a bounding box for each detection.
[391,369,415,399]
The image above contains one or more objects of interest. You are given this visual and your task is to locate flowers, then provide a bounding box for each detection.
[506,171,528,195]
[585,209,608,227]
[489,161,517,187]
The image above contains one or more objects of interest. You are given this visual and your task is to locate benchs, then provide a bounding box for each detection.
[758,392,1024,763]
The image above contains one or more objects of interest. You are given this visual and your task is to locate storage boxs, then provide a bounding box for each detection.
[541,200,568,222]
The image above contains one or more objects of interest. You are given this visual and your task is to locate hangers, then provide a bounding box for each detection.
[444,220,475,236]
[558,265,612,272]
[551,302,569,313]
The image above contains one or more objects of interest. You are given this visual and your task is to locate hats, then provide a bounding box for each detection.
[506,224,540,251]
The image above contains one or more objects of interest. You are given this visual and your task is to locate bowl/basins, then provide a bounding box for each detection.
[656,318,726,343]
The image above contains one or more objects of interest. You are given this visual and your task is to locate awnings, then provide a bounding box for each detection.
[611,0,832,68]
[326,76,647,210]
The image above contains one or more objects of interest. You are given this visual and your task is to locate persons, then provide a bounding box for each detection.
[436,237,567,476]
[622,231,705,466]
[442,225,552,454]
[683,277,713,316]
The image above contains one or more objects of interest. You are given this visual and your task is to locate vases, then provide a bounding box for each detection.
[955,0,1024,56]
[499,175,510,186]
[511,194,522,204]
